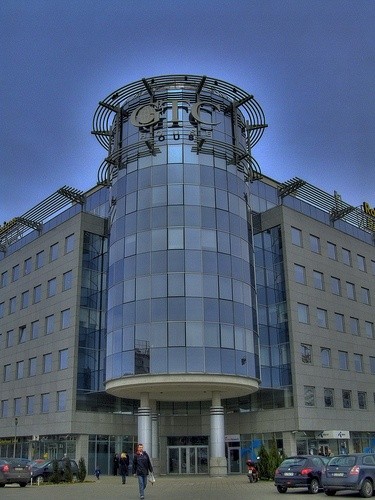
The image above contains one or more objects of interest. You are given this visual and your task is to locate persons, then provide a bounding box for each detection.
[113,451,129,484]
[318,448,334,457]
[61,453,70,481]
[94,466,100,479]
[132,444,154,498]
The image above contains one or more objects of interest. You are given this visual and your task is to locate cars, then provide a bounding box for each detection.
[29,459,80,484]
[0,457,32,488]
[321,453,375,498]
[274,454,331,494]
[16,458,46,474]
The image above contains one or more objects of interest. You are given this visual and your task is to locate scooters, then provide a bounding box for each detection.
[246,457,262,483]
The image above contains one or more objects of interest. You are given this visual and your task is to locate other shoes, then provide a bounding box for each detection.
[140,495,144,499]
[122,482,125,484]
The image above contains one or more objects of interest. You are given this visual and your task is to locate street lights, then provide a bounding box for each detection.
[13,417,19,457]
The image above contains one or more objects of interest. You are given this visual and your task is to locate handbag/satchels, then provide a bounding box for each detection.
[147,472,155,483]
